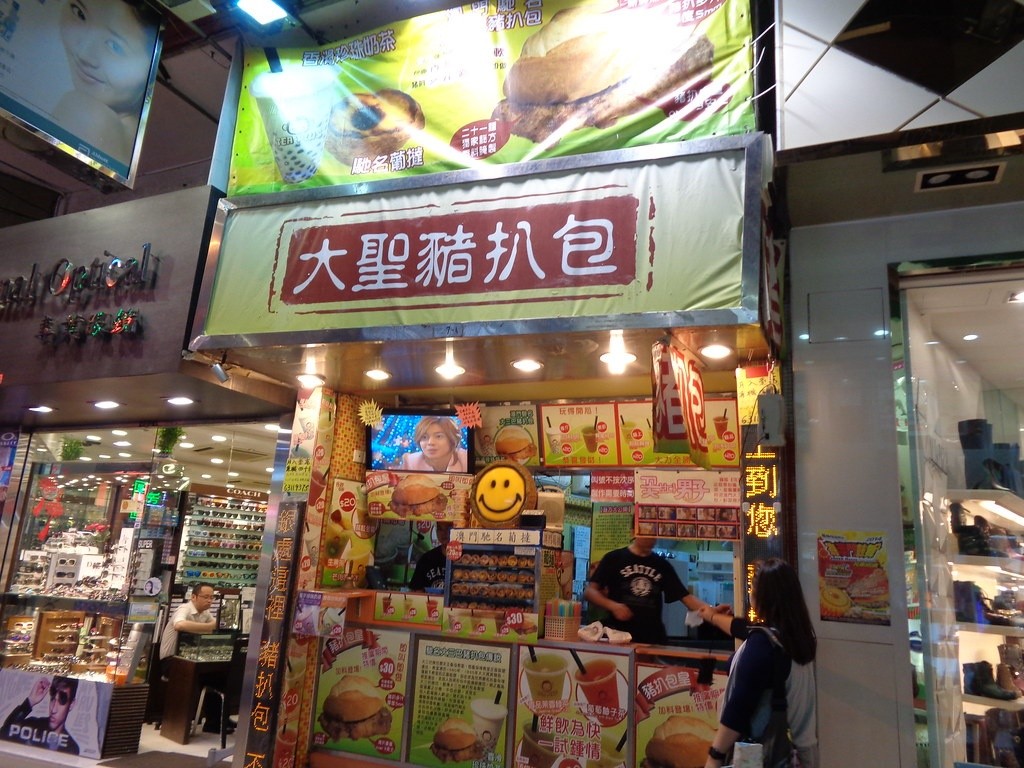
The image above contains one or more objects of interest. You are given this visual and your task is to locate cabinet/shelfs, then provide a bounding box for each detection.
[909,428,1024,768]
[1,460,271,682]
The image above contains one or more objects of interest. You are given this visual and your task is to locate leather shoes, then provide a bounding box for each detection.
[203,721,239,735]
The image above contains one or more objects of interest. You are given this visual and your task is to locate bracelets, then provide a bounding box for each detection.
[710,612,717,624]
[708,745,727,760]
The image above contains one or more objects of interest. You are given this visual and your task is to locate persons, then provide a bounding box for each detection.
[583,513,732,646]
[0,675,80,757]
[401,415,467,472]
[408,521,453,593]
[158,582,216,678]
[697,557,819,768]
[52,0,162,167]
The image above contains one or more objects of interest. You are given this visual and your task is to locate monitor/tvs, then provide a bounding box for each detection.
[366,408,476,476]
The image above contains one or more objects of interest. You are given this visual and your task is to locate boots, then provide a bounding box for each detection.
[949,503,1024,561]
[957,419,1024,500]
[985,708,1024,768]
[954,580,1024,628]
[962,645,1024,701]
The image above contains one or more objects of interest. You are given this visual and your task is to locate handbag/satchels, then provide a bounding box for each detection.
[733,740,764,768]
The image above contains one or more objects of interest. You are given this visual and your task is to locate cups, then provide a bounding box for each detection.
[346,545,372,588]
[621,421,636,443]
[545,427,562,454]
[732,742,763,768]
[297,610,315,635]
[574,658,620,725]
[713,416,730,439]
[304,530,320,566]
[273,727,297,768]
[581,426,598,453]
[447,610,459,630]
[318,608,342,635]
[519,720,564,768]
[403,599,413,615]
[495,618,509,635]
[471,617,481,632]
[521,649,570,714]
[251,64,333,184]
[383,597,391,613]
[426,600,438,617]
[284,661,305,705]
[470,698,508,754]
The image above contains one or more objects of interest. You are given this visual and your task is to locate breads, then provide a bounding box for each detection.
[645,714,718,768]
[433,716,476,751]
[495,428,529,454]
[392,474,440,505]
[502,3,653,105]
[323,674,384,723]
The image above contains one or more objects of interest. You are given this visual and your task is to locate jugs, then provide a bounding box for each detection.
[536,486,565,532]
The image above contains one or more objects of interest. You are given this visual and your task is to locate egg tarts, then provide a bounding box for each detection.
[451,554,535,614]
[323,88,425,167]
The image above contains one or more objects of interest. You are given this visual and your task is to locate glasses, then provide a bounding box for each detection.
[181,499,267,588]
[199,595,214,599]
[179,599,239,662]
[4,622,124,678]
[49,687,69,705]
[15,531,147,603]
